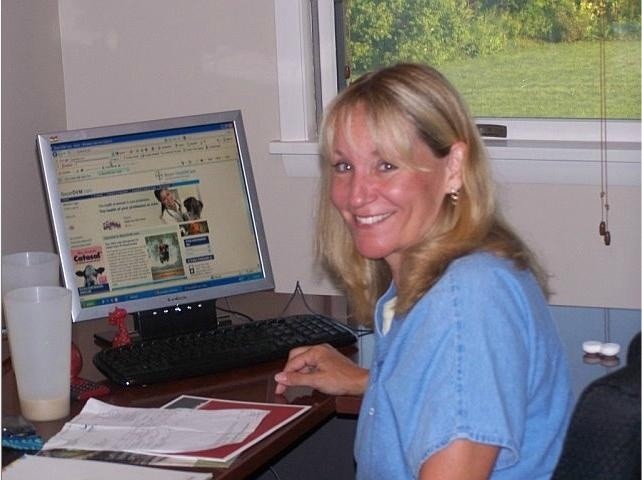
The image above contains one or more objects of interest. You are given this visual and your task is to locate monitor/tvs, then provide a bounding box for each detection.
[36,109,276,346]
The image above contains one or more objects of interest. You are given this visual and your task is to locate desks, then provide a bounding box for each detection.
[2,333,363,480]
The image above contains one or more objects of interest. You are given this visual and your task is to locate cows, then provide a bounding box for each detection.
[76,265,105,287]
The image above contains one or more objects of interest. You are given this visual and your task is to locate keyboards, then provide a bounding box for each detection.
[92,313,359,387]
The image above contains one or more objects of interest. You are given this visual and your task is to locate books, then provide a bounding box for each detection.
[2,394,313,479]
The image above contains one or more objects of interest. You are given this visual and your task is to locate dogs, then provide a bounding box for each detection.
[159,244,169,264]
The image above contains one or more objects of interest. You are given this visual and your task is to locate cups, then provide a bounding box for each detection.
[1,250,60,285]
[2,285,72,424]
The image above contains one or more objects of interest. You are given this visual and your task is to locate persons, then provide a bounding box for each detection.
[159,240,169,262]
[273,62,574,480]
[154,188,189,225]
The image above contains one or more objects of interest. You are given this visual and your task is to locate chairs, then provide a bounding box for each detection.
[548,330,641,480]
